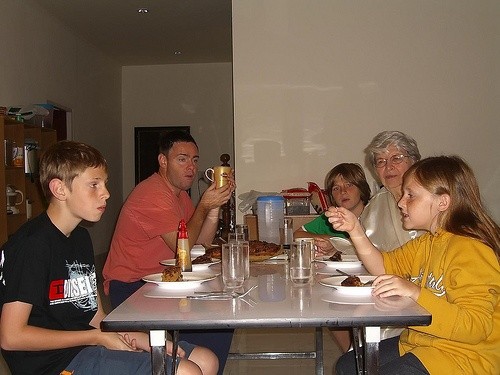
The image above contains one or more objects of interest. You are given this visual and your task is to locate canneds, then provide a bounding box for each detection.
[11,147,23,167]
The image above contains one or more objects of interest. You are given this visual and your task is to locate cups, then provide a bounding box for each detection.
[6,184,24,214]
[12,147,25,168]
[221,244,245,288]
[290,241,312,287]
[205,166,230,189]
[235,224,248,241]
[228,233,245,243]
[230,241,251,280]
[295,236,315,262]
[278,218,293,251]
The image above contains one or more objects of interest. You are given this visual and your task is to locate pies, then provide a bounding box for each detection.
[191,253,212,264]
[329,251,343,262]
[161,265,182,282]
[205,239,281,262]
[340,275,364,286]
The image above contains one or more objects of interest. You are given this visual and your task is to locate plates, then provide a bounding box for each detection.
[314,258,362,268]
[141,271,217,290]
[317,275,378,295]
[160,257,221,270]
[321,290,376,305]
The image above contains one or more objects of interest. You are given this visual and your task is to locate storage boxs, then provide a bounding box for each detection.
[284,192,313,214]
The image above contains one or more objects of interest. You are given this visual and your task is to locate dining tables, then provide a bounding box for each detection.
[101,261,432,375]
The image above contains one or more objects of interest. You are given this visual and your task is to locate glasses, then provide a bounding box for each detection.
[375,154,410,168]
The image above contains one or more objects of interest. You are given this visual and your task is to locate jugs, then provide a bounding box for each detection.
[256,195,285,245]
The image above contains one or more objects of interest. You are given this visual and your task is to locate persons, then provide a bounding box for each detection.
[0,141,220,375]
[293,163,371,354]
[324,154,500,375]
[353,131,428,341]
[103,132,235,375]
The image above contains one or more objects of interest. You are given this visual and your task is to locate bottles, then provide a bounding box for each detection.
[176,217,192,272]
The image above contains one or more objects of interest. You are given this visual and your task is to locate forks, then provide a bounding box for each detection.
[186,284,259,302]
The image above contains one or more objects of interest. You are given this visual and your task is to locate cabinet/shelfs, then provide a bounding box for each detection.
[0,110,57,247]
